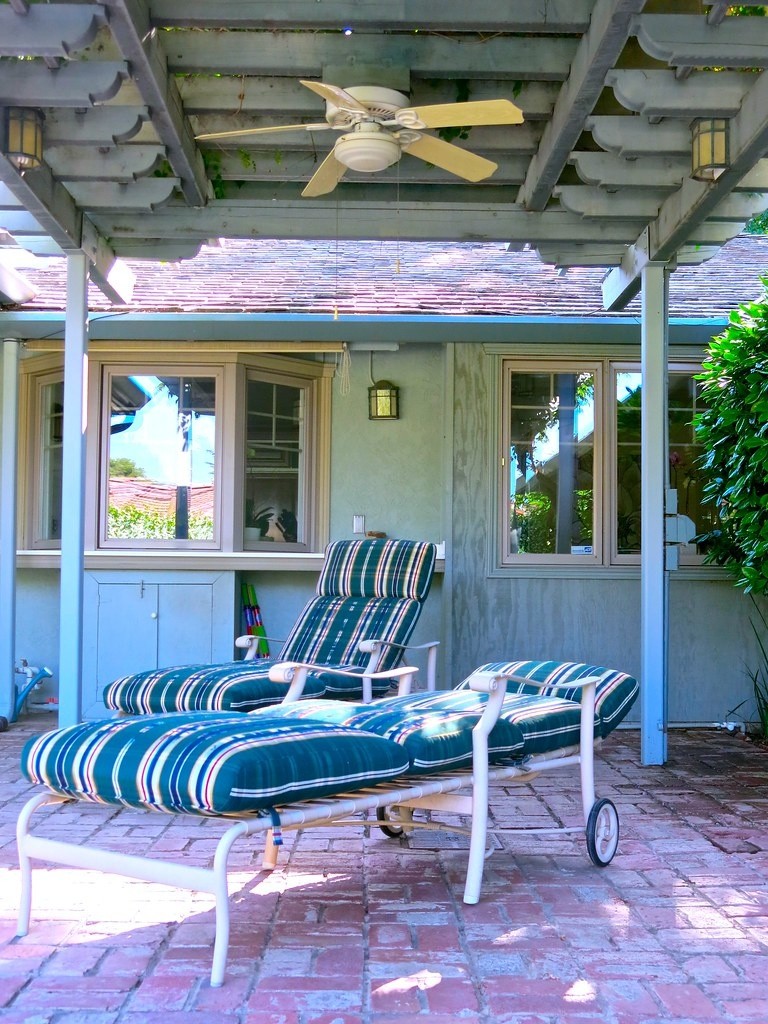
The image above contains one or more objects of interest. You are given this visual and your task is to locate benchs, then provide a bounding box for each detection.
[16,658,640,984]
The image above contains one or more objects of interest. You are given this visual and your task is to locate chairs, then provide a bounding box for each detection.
[100,538,438,839]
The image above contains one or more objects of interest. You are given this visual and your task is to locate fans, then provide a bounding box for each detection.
[193,80,525,198]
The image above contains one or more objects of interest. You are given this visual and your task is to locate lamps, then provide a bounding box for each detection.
[3,106,46,176]
[690,117,729,185]
[368,380,400,421]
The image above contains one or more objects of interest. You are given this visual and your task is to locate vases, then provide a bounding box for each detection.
[244,528,261,541]
[678,543,698,554]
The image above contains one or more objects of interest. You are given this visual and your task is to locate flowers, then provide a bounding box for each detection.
[246,448,274,528]
[670,452,686,513]
